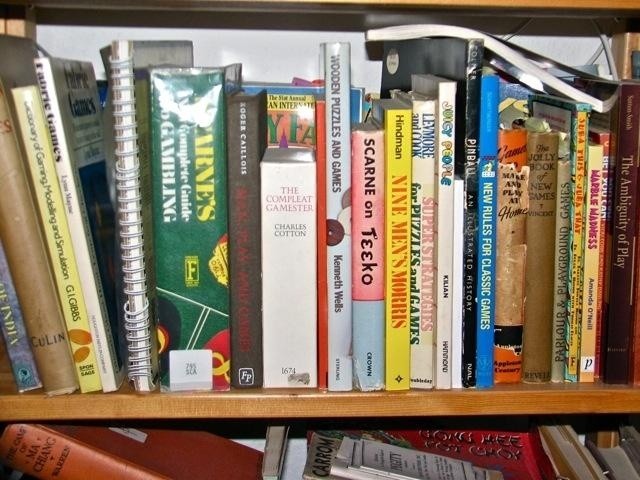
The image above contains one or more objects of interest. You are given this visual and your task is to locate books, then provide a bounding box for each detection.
[1,20,640,394]
[1,424,609,480]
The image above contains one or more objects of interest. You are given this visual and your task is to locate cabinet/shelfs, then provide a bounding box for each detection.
[0,1,640,418]
